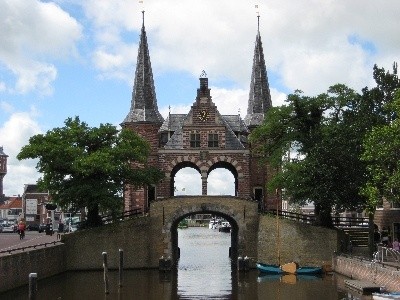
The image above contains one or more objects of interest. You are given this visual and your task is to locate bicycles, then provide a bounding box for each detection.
[18,225,27,240]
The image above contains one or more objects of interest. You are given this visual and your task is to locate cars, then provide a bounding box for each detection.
[0,217,50,233]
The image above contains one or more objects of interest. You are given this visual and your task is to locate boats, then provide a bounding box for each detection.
[218,221,231,232]
[256,261,323,275]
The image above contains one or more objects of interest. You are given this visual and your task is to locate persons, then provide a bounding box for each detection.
[18,221,25,239]
[393,239,400,263]
